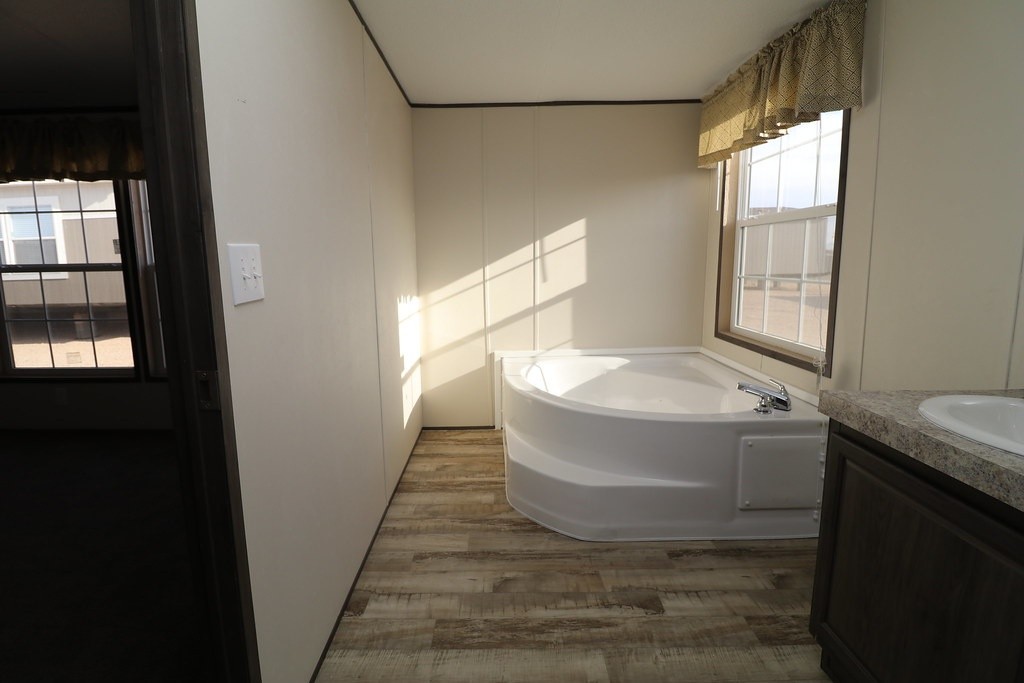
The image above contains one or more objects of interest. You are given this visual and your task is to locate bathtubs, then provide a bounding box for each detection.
[499,351,831,543]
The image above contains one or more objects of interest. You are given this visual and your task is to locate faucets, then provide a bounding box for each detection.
[736,379,792,411]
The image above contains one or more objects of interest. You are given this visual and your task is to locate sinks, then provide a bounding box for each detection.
[918,394,1024,455]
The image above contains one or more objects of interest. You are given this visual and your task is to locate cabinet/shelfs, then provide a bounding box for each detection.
[808,420,1024,683]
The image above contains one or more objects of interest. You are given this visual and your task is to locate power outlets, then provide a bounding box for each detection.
[227,243,265,305]
